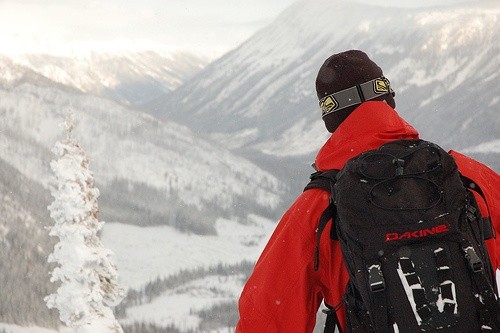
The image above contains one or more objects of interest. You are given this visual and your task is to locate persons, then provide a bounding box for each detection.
[235,50,500,333]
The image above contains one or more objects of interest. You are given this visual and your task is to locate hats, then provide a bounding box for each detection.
[316,50,396,133]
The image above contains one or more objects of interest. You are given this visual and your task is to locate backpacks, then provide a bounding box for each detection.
[303,139,500,333]
